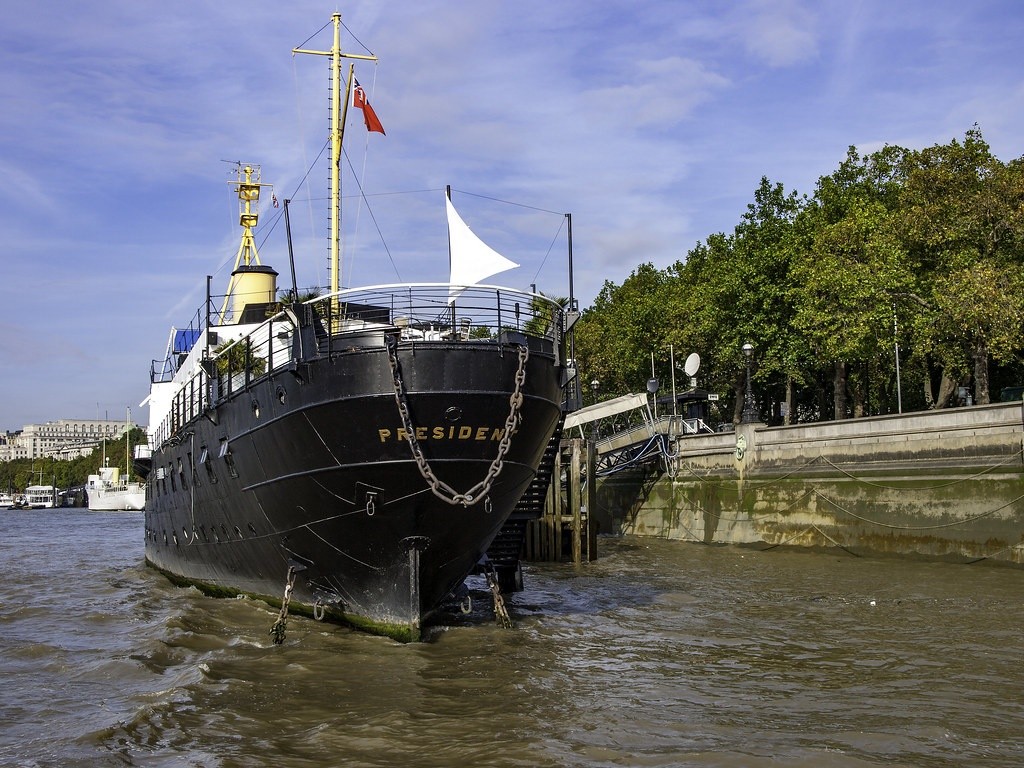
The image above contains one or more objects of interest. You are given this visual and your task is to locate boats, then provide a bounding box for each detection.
[138,5,577,643]
[0,467,74,510]
[85,406,147,510]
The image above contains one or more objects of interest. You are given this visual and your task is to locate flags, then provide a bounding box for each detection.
[353,75,386,136]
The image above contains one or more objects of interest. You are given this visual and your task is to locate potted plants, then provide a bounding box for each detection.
[213,334,267,395]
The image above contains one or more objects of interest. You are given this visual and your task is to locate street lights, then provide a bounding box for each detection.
[590,377,601,438]
[741,340,761,423]
